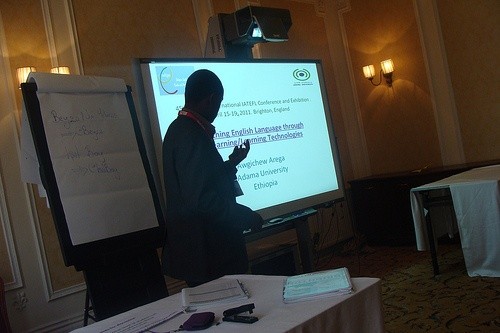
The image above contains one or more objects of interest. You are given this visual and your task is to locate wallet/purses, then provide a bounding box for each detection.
[179,312,215,331]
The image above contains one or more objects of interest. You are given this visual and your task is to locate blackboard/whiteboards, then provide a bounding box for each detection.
[21,82,172,267]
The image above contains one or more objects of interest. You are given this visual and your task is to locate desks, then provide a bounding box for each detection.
[67,275,384,333]
[410,164,500,280]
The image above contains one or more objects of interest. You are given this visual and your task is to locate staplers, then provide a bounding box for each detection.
[222,303,258,324]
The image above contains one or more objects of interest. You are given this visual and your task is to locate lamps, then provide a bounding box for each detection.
[363,59,394,88]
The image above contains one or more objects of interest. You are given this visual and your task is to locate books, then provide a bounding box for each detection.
[182,279,249,308]
[282,267,353,304]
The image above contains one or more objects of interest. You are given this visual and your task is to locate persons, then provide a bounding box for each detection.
[162,69,265,288]
